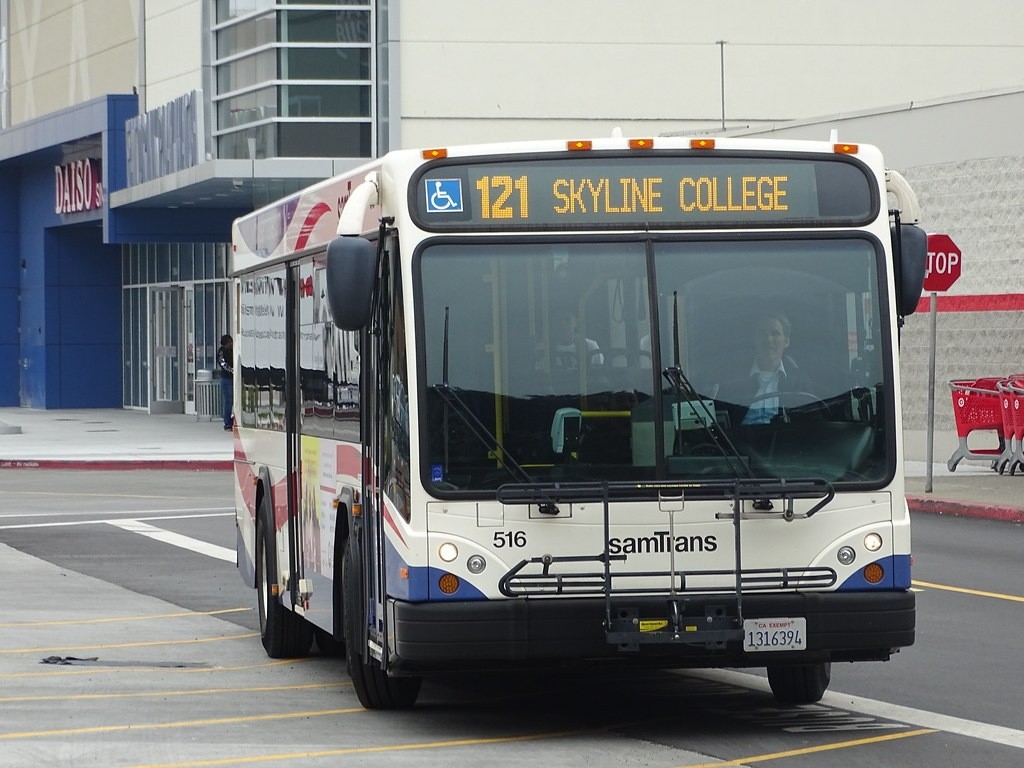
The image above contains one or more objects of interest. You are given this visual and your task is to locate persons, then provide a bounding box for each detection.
[217,335,234,431]
[607,386,637,410]
[542,308,604,366]
[710,312,815,424]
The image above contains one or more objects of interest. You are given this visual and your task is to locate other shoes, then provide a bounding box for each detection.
[223,421,233,432]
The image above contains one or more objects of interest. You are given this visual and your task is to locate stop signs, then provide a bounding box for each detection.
[921,233,961,294]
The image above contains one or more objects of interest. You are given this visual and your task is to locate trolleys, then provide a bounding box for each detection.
[947,372,1024,474]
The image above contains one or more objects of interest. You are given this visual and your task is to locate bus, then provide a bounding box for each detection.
[228,138,923,706]
